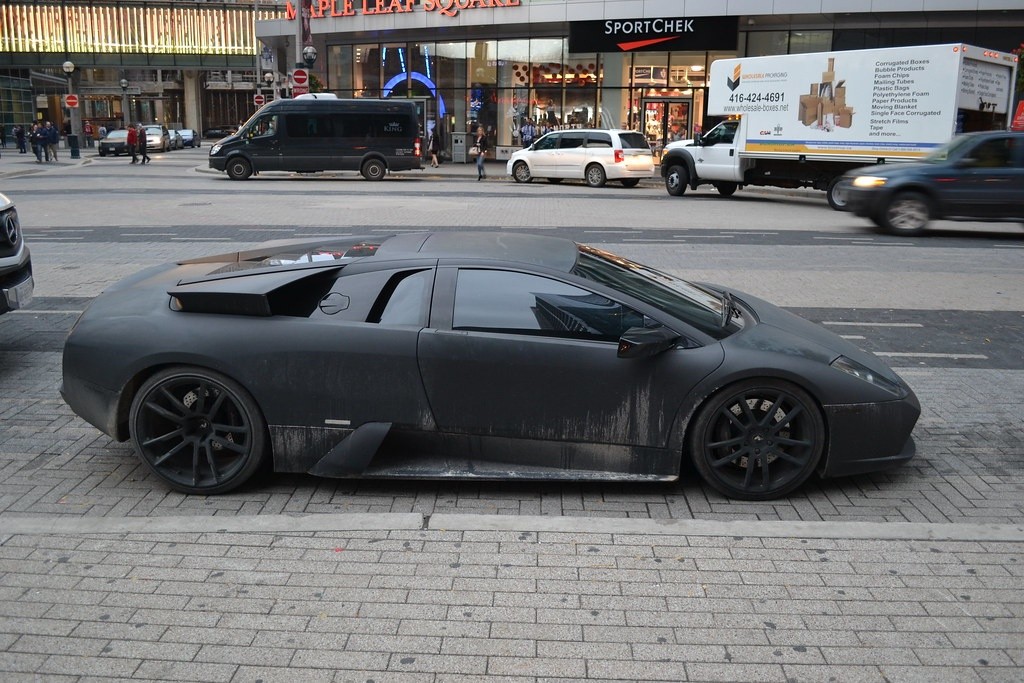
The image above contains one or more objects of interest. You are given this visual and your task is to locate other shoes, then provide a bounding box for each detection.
[147,159,151,163]
[141,162,145,164]
[129,161,135,164]
[435,165,439,168]
[430,165,433,167]
[19,152,26,153]
[137,159,139,162]
[36,159,42,163]
[46,161,50,163]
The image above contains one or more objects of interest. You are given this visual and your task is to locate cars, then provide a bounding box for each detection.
[98,125,201,157]
[658,41,1018,197]
[506,128,656,189]
[54,235,921,503]
[837,129,1024,237]
[0,191,37,318]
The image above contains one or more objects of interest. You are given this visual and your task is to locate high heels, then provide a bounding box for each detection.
[478,175,482,181]
[483,175,486,179]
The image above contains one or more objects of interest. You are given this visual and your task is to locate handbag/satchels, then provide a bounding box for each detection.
[468,143,479,155]
[425,135,433,151]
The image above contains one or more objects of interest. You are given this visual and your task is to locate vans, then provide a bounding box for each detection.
[209,98,425,181]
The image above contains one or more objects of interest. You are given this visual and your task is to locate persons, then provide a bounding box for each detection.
[519,118,537,149]
[473,127,489,181]
[83,121,152,164]
[0,121,68,164]
[542,121,555,136]
[237,119,274,139]
[418,122,441,167]
[646,113,675,156]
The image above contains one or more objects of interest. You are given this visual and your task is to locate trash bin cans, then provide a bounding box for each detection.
[452,132,475,164]
